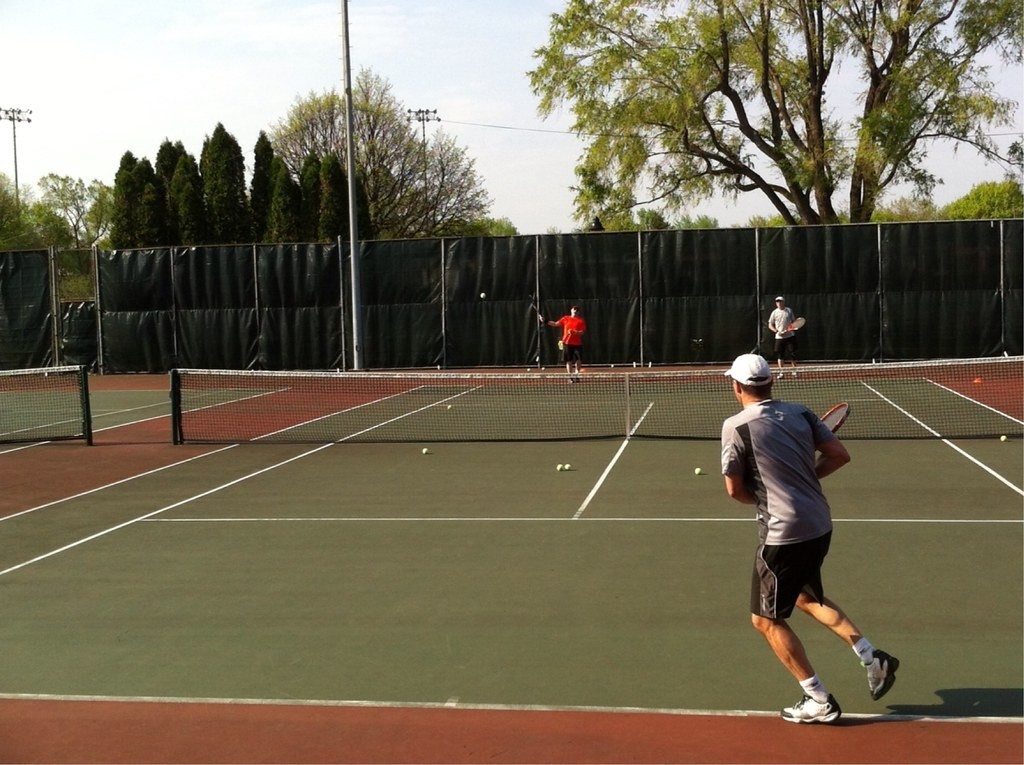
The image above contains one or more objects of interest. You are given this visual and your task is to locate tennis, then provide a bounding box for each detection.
[422,447,429,454]
[526,366,546,372]
[610,364,615,368]
[565,464,571,471]
[447,404,453,410]
[556,463,564,472]
[694,466,702,476]
[480,292,486,298]
[1000,435,1008,442]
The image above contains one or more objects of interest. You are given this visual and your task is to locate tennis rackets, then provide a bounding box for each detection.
[817,402,851,433]
[781,317,806,334]
[529,295,542,318]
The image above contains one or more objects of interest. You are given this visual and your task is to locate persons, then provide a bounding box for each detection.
[721,354,899,723]
[768,296,798,379]
[538,306,586,384]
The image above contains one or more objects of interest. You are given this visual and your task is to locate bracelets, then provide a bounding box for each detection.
[544,319,548,324]
[774,330,778,334]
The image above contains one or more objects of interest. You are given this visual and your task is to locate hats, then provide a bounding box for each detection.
[724,354,772,386]
[572,306,580,308]
[775,297,784,301]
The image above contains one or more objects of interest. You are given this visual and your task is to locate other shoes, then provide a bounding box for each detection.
[576,378,580,382]
[792,372,799,377]
[777,374,784,379]
[570,379,574,383]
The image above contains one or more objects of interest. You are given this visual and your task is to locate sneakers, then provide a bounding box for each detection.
[866,649,899,701]
[781,690,842,724]
